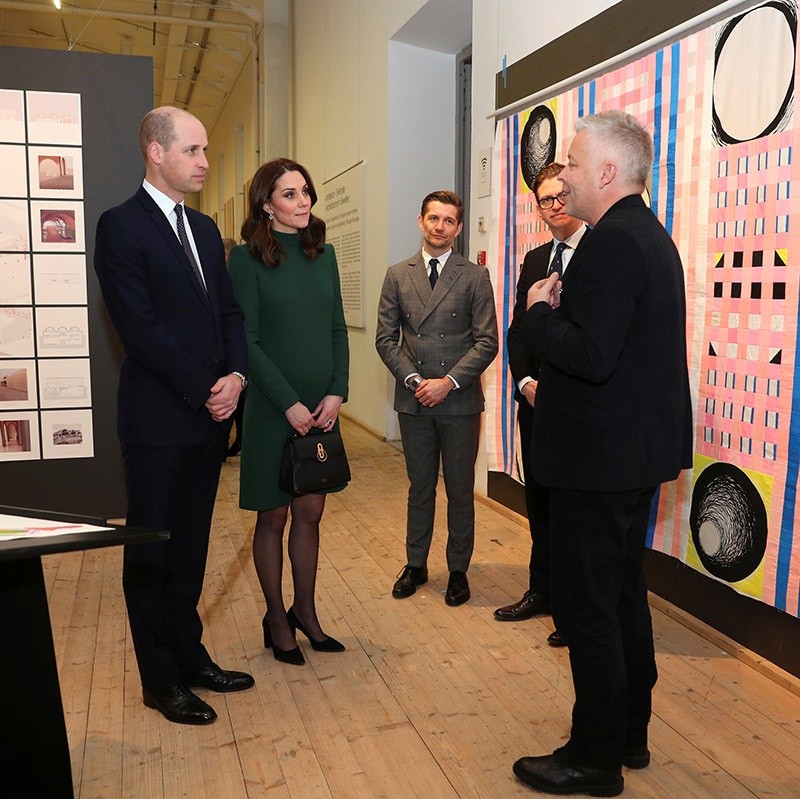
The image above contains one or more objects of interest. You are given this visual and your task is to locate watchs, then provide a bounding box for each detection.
[231,371,248,389]
[407,375,423,392]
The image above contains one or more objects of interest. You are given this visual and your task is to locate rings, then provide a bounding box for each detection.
[327,419,333,426]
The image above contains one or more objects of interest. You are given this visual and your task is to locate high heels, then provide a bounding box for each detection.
[288,606,345,651]
[262,611,305,665]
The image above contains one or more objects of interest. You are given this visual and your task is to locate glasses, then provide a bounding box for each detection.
[538,192,566,210]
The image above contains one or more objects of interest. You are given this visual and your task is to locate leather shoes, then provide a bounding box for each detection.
[143,684,217,725]
[185,662,255,693]
[623,742,651,769]
[548,631,566,645]
[493,589,552,620]
[392,565,428,599]
[445,571,470,605]
[513,747,624,797]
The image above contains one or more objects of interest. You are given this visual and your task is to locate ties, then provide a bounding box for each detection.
[428,258,439,290]
[548,243,567,279]
[173,204,211,308]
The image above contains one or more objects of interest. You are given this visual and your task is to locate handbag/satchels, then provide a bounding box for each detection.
[280,425,351,498]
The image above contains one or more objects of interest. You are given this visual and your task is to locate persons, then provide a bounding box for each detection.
[93,105,255,725]
[227,157,351,665]
[222,237,245,463]
[374,190,499,607]
[493,162,594,648]
[512,109,693,798]
